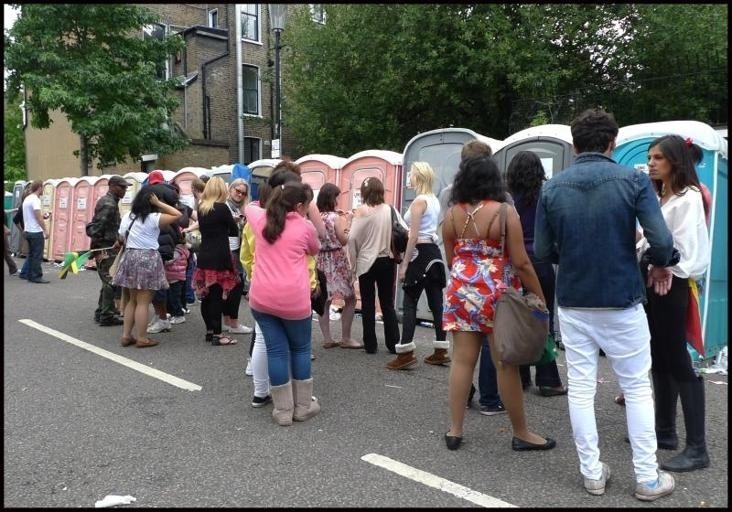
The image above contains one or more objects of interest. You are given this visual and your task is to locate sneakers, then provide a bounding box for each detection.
[583,462,611,496]
[634,470,676,502]
[251,396,271,408]
[12,269,51,284]
[466,397,507,416]
[95,297,254,376]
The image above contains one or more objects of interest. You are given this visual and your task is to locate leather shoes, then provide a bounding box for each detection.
[511,434,557,451]
[444,429,463,451]
[323,340,398,354]
[661,376,710,473]
[624,376,678,450]
[521,379,625,406]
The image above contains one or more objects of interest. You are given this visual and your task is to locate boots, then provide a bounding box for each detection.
[385,341,418,370]
[423,340,452,365]
[270,379,295,426]
[292,375,321,422]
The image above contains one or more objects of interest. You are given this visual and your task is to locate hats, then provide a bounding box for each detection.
[108,175,134,187]
[148,171,165,185]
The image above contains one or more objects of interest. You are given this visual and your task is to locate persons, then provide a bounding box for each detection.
[4,211,23,277]
[11,182,33,280]
[22,180,52,284]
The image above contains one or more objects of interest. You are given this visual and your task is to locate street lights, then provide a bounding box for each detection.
[265,3,288,159]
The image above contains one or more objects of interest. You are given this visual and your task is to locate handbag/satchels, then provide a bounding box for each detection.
[388,204,409,264]
[491,286,551,367]
[310,268,328,318]
[108,245,125,278]
